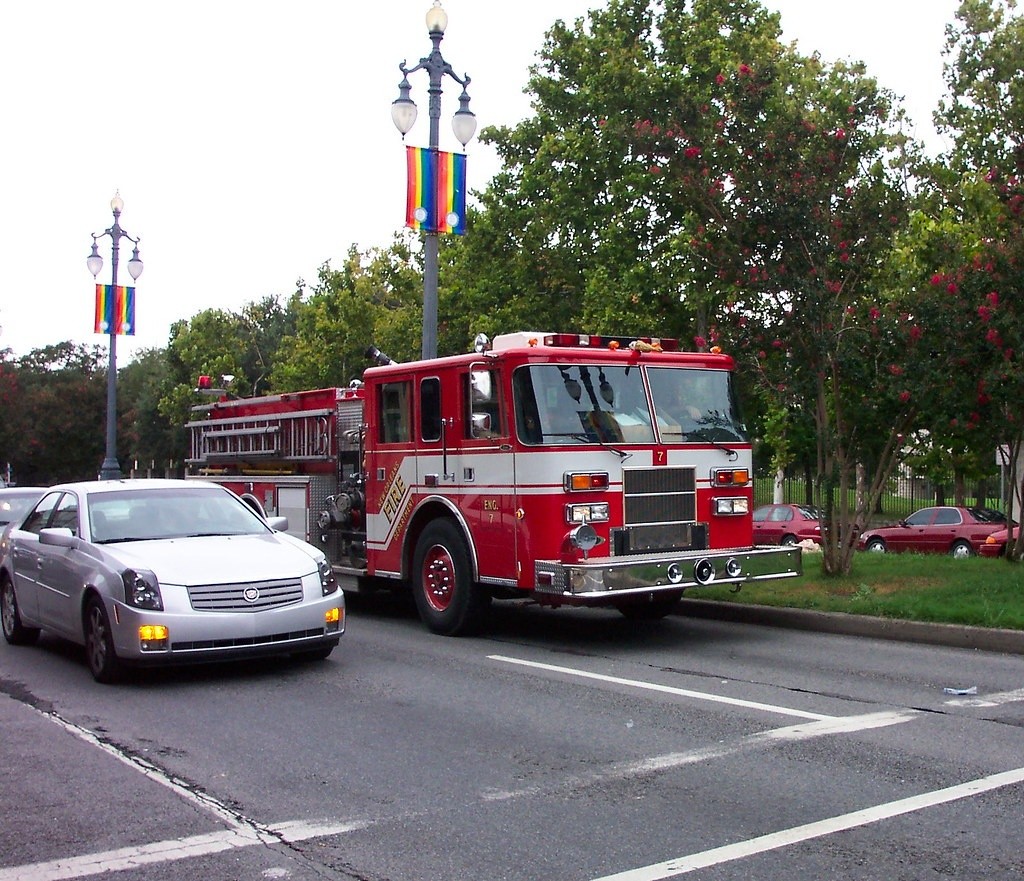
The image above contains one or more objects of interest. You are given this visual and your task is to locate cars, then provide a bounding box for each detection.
[0,487,49,546]
[752,503,860,549]
[0,477,348,686]
[854,505,1024,561]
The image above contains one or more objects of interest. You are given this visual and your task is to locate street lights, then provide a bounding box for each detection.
[390,0,481,361]
[86,186,145,481]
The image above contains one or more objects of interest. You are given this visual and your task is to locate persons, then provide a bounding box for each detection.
[667,376,701,422]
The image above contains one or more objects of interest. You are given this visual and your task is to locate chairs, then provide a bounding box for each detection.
[92,511,112,539]
[128,507,157,537]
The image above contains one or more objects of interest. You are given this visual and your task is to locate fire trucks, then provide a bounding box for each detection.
[182,329,804,640]
